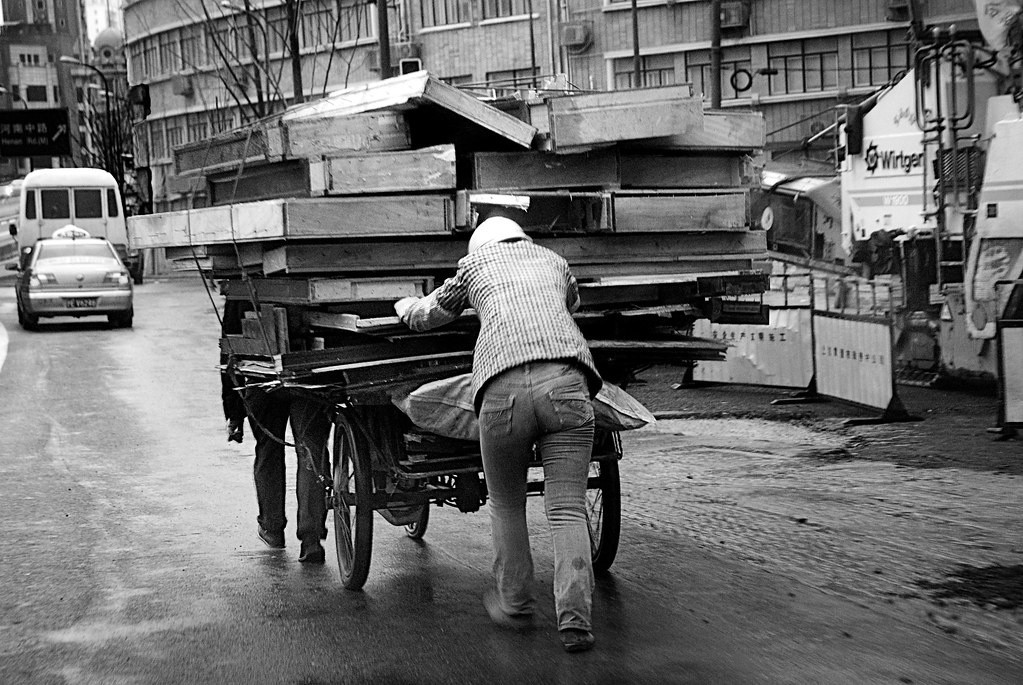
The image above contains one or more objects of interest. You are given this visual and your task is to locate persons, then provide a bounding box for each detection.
[220,302,331,562]
[394,216,603,649]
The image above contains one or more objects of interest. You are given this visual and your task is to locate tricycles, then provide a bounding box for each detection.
[321,393,621,591]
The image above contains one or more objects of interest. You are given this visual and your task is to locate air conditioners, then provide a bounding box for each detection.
[365,50,382,71]
[561,25,584,45]
[800,109,836,139]
[710,1,749,30]
[395,40,417,61]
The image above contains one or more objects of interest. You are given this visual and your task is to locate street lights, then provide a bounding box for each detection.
[0,87,34,172]
[222,2,271,117]
[60,56,117,174]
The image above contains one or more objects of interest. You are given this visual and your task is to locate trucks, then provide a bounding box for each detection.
[838,41,1023,282]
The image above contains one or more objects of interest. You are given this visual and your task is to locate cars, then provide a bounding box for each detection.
[4,225,134,331]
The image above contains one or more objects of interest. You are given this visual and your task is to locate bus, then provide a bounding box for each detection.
[8,168,132,279]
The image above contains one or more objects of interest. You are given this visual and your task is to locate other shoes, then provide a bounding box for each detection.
[258,524,287,550]
[500,606,534,631]
[296,540,326,562]
[559,628,595,653]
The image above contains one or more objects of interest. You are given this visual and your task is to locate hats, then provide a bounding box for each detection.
[466,215,535,253]
